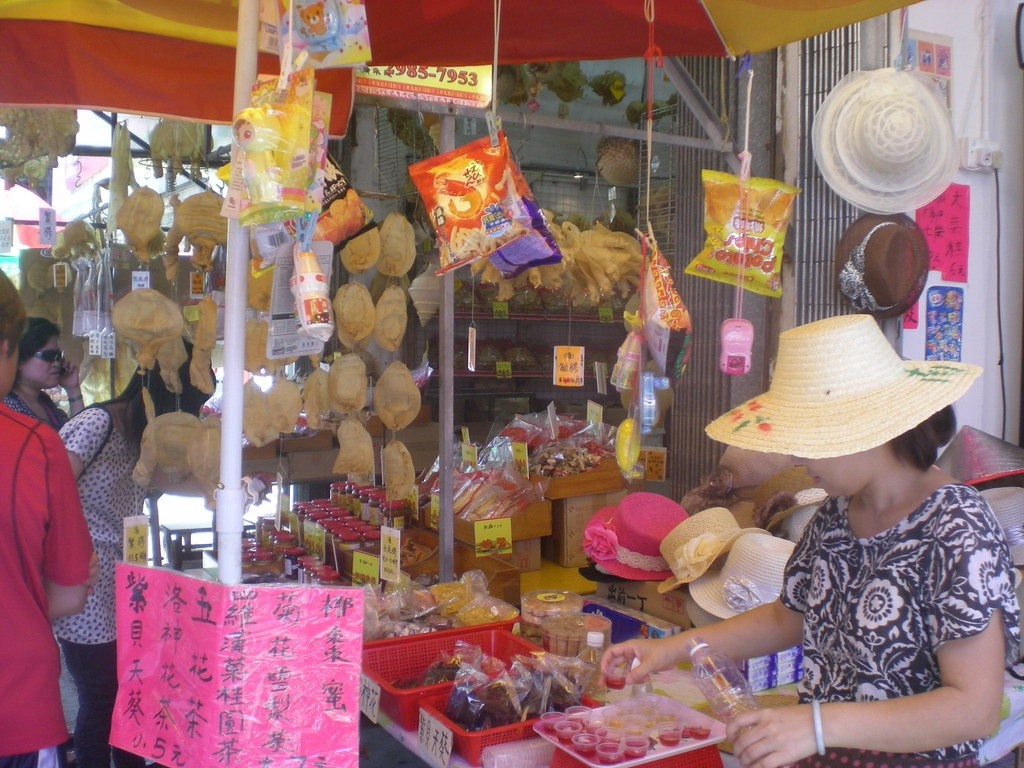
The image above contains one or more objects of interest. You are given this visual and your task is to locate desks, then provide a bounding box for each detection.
[377,668,1024,768]
[519,557,597,596]
[143,475,378,566]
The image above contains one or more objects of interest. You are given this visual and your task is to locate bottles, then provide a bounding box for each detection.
[688,638,762,738]
[241,480,405,585]
[576,632,610,693]
[628,659,650,695]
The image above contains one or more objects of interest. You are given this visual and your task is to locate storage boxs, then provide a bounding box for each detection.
[245,404,695,630]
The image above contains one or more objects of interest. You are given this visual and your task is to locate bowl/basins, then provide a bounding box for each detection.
[541,694,714,764]
[606,667,627,689]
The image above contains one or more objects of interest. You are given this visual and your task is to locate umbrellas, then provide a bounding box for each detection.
[0,0,925,586]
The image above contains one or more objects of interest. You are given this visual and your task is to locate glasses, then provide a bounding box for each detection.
[36,349,65,361]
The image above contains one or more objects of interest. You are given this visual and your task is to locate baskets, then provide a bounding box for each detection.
[362,615,724,768]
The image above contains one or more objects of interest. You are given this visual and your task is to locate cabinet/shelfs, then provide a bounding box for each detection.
[405,161,637,423]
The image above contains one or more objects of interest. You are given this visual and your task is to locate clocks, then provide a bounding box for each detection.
[489,391,536,424]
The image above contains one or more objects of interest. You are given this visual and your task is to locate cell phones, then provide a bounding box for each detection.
[60,367,68,375]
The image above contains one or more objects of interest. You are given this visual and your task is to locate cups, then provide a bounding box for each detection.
[482,737,555,768]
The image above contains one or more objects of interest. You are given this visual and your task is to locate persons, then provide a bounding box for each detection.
[600,313,1022,768]
[0,267,216,768]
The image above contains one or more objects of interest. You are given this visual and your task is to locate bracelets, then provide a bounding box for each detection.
[812,699,825,756]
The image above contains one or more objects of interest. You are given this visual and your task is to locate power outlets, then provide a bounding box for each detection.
[961,138,1002,172]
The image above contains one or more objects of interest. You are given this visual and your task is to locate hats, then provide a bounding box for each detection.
[813,67,958,216]
[836,211,931,318]
[982,487,1024,567]
[933,424,1024,486]
[584,470,827,620]
[704,312,984,460]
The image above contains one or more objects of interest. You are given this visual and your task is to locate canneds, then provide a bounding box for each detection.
[242,480,408,585]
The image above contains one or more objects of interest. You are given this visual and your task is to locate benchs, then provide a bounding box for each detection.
[159,518,253,573]
